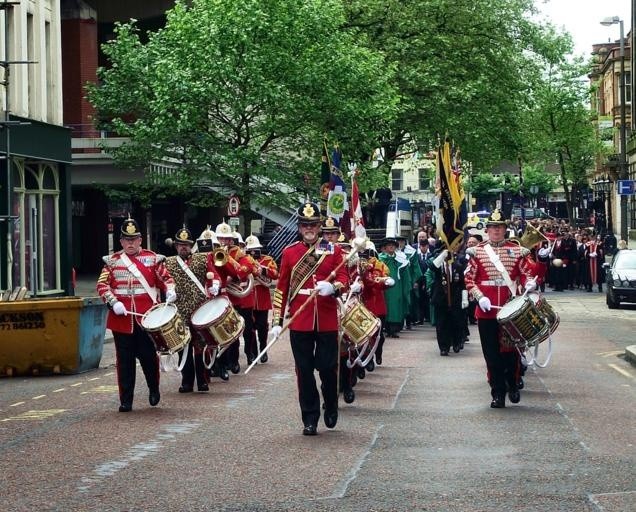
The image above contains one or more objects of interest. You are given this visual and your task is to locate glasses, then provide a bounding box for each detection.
[302,223,316,227]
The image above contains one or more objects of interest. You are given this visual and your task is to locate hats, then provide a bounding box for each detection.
[120,212,142,240]
[295,193,320,225]
[382,235,408,248]
[174,223,194,245]
[419,239,429,245]
[486,202,508,228]
[320,214,340,233]
[365,240,378,255]
[201,217,263,250]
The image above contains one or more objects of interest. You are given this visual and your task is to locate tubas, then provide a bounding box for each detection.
[245,252,272,287]
[509,222,549,249]
[214,243,254,298]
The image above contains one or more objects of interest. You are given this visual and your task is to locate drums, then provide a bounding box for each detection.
[190,295,245,350]
[141,303,192,353]
[527,292,559,344]
[495,295,549,347]
[340,295,380,347]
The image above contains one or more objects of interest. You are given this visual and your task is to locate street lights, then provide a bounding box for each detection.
[599,9,628,247]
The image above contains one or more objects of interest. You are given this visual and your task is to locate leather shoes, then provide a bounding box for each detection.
[441,322,477,356]
[119,402,132,412]
[304,351,382,435]
[179,353,268,393]
[382,318,430,338]
[149,384,160,406]
[491,395,505,408]
[541,284,602,292]
[508,382,520,403]
[515,377,524,389]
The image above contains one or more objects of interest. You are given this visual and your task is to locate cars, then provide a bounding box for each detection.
[601,241,636,311]
[465,212,495,226]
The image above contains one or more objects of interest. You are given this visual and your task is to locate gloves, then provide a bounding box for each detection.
[524,280,537,292]
[433,249,449,269]
[478,296,491,313]
[314,281,336,297]
[349,281,362,293]
[113,301,127,316]
[462,289,470,309]
[268,326,282,340]
[165,284,176,303]
[385,278,395,286]
[208,280,220,296]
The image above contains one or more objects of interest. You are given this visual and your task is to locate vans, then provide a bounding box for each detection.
[512,203,556,227]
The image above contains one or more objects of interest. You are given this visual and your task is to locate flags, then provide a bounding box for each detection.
[315,135,366,247]
[431,128,468,251]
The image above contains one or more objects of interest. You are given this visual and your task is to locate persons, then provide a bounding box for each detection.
[194,224,241,380]
[214,217,252,373]
[243,229,278,363]
[97,212,176,412]
[272,203,350,435]
[466,206,542,409]
[164,223,222,393]
[381,229,481,355]
[319,213,394,407]
[232,227,263,367]
[517,215,609,291]
